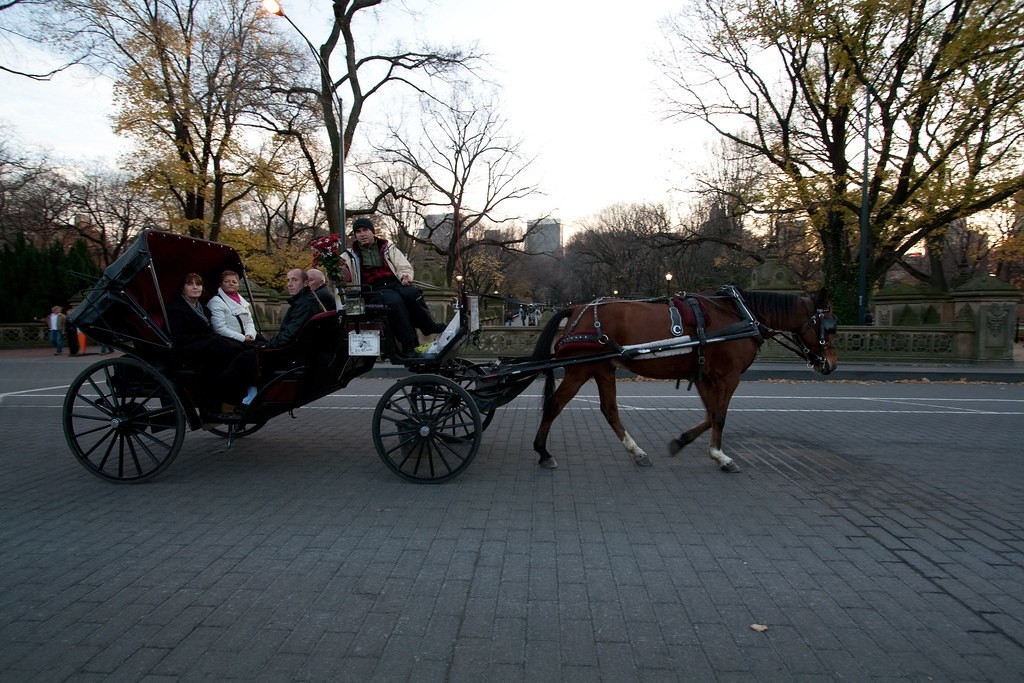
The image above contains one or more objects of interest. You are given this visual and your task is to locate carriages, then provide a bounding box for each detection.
[61,225,840,487]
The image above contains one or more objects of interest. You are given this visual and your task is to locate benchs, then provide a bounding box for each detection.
[339,282,391,321]
[262,309,341,366]
[135,278,213,373]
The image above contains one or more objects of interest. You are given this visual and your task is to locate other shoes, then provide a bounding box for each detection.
[68,353,77,357]
[53,352,62,355]
[106,350,114,354]
[221,402,234,413]
[423,322,447,336]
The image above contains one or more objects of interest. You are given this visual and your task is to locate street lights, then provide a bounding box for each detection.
[261,0,347,246]
[456,275,463,305]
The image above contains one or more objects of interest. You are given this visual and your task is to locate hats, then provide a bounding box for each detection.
[353,218,374,231]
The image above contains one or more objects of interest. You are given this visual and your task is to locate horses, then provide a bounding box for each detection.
[534,288,837,472]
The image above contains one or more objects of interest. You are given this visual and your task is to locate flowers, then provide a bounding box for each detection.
[301,232,346,304]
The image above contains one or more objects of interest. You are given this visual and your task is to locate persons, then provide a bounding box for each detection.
[47,306,66,355]
[339,218,447,358]
[508,310,513,326]
[520,305,564,326]
[65,309,86,357]
[100,345,114,354]
[152,268,336,414]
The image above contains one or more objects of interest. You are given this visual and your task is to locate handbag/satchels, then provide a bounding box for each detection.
[250,334,268,346]
